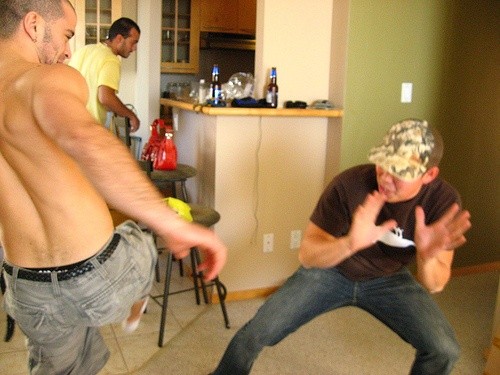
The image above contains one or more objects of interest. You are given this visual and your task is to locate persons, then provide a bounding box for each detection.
[208,119,471,375]
[0,0,227,375]
[68,18,141,132]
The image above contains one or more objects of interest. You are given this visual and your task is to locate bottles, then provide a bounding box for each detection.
[166,30,172,40]
[208,64,222,106]
[265,68,278,108]
[197,79,207,105]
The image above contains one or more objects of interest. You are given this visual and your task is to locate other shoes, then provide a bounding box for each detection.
[121,295,149,330]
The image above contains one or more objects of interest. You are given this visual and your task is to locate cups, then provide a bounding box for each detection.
[168,83,190,98]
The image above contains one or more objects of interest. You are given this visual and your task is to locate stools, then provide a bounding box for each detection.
[144,203,233,347]
[138,158,196,284]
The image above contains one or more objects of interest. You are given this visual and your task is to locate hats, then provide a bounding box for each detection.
[369,120,445,181]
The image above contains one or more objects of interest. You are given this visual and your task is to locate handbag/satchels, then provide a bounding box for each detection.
[141,120,177,170]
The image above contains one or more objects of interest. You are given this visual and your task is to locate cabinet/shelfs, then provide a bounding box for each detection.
[73,0,122,53]
[161,0,201,73]
[200,0,257,32]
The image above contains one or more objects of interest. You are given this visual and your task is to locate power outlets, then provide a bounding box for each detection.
[291,231,302,249]
[264,233,275,253]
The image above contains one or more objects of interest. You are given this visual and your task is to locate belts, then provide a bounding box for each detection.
[3,233,121,282]
